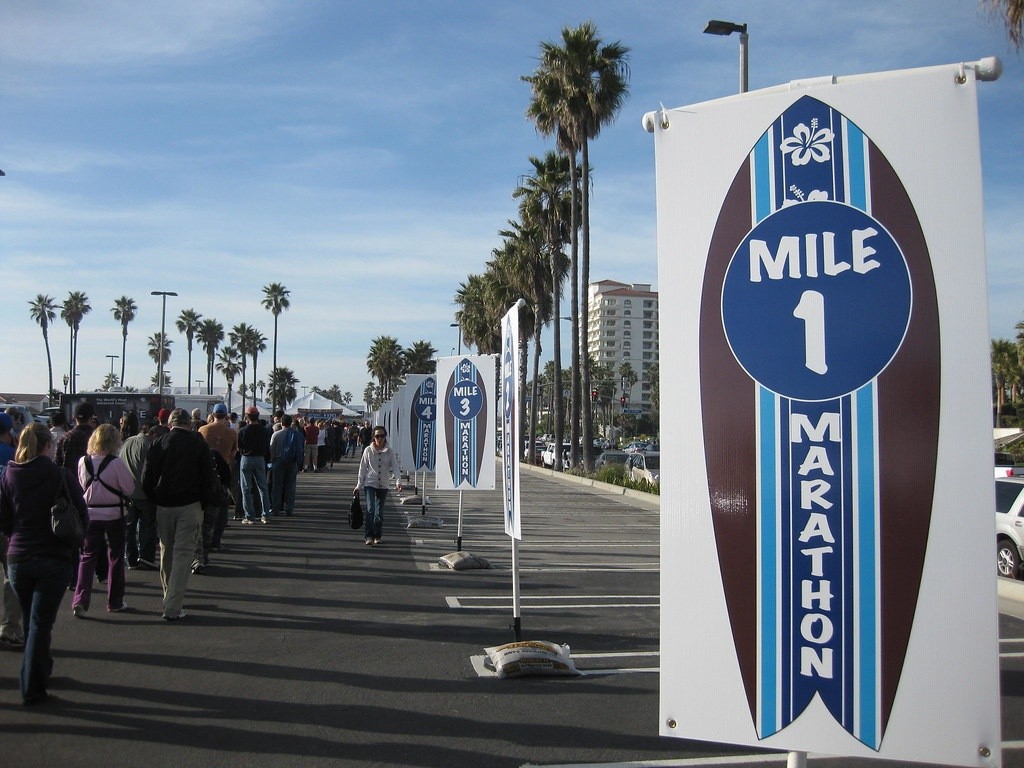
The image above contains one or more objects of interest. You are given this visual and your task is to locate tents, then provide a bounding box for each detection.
[232,391,362,419]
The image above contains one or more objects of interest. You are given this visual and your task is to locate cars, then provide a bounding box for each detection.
[995,476,1024,582]
[522,434,660,491]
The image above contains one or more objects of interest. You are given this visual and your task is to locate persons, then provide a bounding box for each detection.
[350,421,372,459]
[0,402,238,699]
[237,405,349,523]
[353,426,402,544]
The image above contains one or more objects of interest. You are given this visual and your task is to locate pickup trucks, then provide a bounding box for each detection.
[994,451,1024,479]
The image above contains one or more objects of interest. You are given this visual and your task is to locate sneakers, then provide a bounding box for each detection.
[0,629,25,647]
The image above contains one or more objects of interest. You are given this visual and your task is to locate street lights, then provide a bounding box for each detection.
[195,380,205,388]
[63,374,70,394]
[151,290,177,398]
[106,355,119,388]
[703,19,752,98]
[163,371,170,388]
[450,323,462,355]
[300,385,309,398]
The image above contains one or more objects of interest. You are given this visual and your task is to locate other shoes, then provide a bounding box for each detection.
[269,508,292,515]
[128,562,137,570]
[365,537,373,545]
[136,557,160,568]
[23,685,49,706]
[97,575,108,583]
[190,561,205,575]
[73,602,86,617]
[68,583,77,591]
[164,605,186,619]
[107,601,128,611]
[297,462,328,473]
[232,514,245,521]
[374,537,383,544]
[261,515,269,524]
[241,517,256,525]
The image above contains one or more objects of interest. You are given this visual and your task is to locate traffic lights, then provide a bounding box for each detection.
[621,396,625,407]
[593,391,599,403]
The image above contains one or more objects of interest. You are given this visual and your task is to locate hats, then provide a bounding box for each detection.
[0,412,14,428]
[74,402,94,419]
[158,409,171,420]
[213,403,228,415]
[245,405,258,415]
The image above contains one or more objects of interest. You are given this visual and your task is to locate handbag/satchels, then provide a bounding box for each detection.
[50,467,85,536]
[347,492,364,529]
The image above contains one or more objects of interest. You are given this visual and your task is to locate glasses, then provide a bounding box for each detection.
[373,434,386,438]
